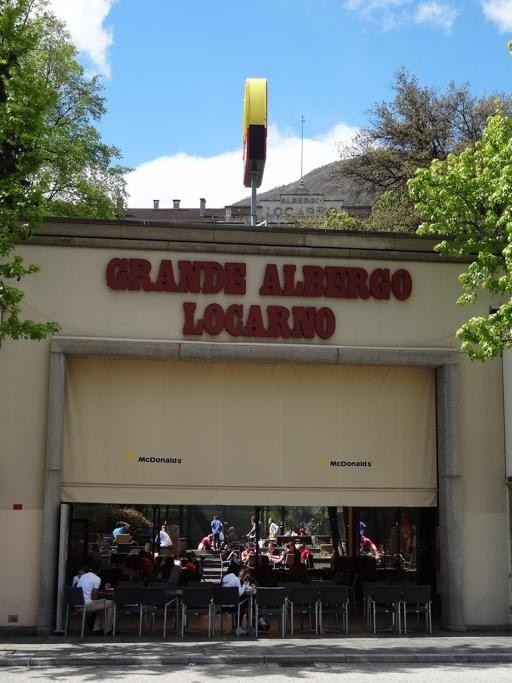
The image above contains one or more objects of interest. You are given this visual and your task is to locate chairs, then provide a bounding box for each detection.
[65,533,435,637]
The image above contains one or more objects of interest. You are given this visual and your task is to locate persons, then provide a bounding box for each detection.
[238,563,271,632]
[71,565,88,587]
[73,561,121,634]
[121,511,391,587]
[112,519,130,543]
[213,561,250,637]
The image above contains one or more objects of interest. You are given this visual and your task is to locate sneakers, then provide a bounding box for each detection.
[236,627,255,635]
[94,629,120,636]
[259,618,267,625]
